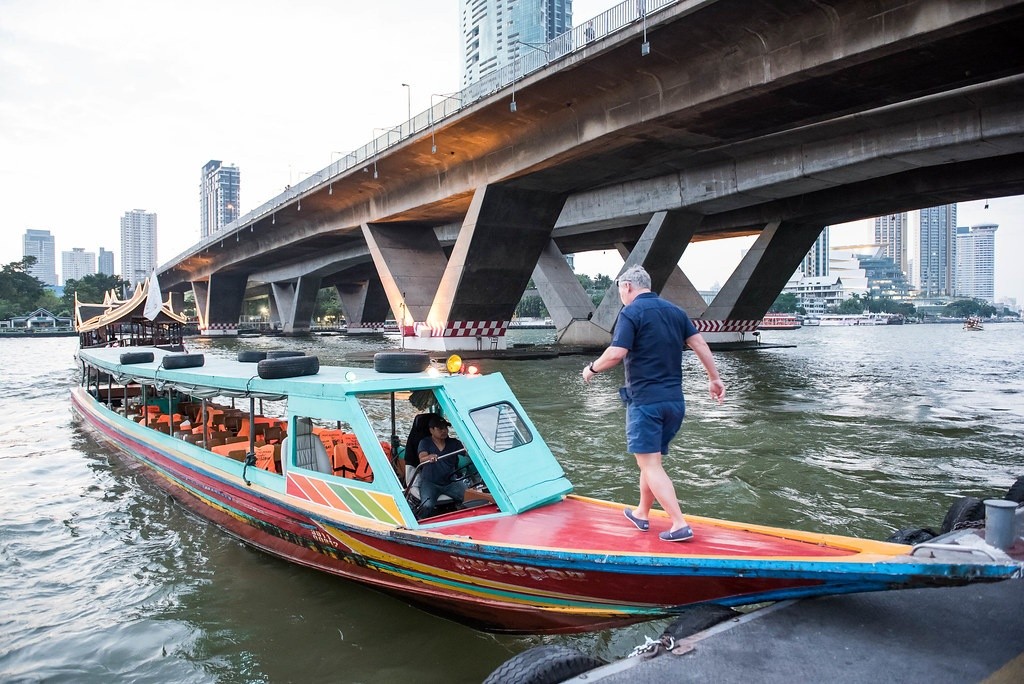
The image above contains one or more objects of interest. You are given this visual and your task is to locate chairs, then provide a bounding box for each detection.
[404,412,454,505]
[136,402,406,484]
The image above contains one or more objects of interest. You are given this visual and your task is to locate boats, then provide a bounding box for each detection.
[963,317,985,331]
[67,345,1024,634]
[73,267,185,353]
[755,310,922,330]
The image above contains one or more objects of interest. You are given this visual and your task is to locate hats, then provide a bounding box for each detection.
[429,416,451,428]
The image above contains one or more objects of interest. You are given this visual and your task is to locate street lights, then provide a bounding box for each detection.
[401,83,411,136]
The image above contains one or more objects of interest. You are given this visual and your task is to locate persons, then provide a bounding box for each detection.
[418,417,470,520]
[582,265,725,541]
[108,333,118,346]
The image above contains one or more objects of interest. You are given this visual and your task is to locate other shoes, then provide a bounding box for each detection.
[659,526,695,542]
[624,509,650,531]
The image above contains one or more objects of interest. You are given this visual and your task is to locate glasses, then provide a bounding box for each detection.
[617,280,630,286]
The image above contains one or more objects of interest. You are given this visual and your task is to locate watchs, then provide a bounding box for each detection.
[588,361,598,374]
